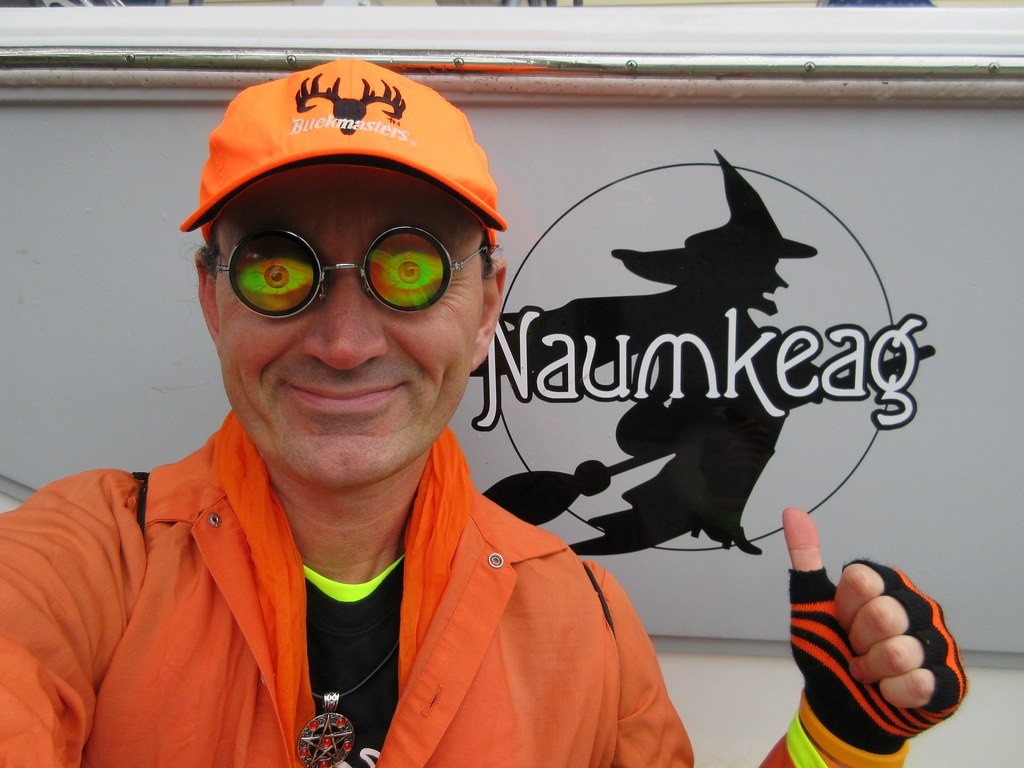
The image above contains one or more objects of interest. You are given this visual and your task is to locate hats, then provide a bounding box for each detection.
[180,59,508,254]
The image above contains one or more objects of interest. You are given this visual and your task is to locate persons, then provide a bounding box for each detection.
[0,54,968,768]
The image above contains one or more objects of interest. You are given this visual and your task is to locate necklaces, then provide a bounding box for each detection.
[296,639,399,768]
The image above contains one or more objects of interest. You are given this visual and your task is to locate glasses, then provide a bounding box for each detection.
[204,225,488,319]
